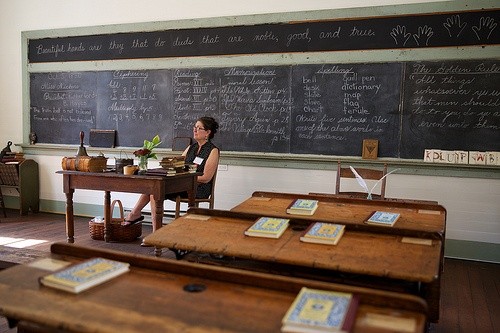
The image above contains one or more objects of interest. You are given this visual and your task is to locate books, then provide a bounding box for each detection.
[39,256,131,295]
[244,216,290,239]
[1,150,26,164]
[285,197,320,215]
[363,209,401,227]
[299,220,347,245]
[136,153,199,176]
[278,284,359,333]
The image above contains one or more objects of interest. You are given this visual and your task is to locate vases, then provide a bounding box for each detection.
[137,155,148,171]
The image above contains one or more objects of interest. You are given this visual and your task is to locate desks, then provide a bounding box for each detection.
[0,192,447,333]
[55,170,205,258]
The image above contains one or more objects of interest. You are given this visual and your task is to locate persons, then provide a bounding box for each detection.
[119,116,220,248]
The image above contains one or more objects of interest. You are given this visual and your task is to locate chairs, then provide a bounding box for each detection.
[336,157,388,198]
[173,152,220,222]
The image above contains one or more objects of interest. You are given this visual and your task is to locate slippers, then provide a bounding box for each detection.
[120,215,145,227]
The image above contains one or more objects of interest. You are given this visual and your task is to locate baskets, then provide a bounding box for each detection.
[89,199,142,240]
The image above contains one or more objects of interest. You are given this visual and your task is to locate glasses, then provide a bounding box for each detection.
[193,126,206,131]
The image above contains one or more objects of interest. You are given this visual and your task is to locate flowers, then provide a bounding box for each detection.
[134,135,164,158]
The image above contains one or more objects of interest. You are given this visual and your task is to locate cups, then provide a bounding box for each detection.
[123,166,134,175]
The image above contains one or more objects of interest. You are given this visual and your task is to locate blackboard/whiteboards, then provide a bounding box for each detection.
[21,1,500,181]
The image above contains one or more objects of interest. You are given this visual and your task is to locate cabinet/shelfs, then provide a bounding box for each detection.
[0,159,39,217]
